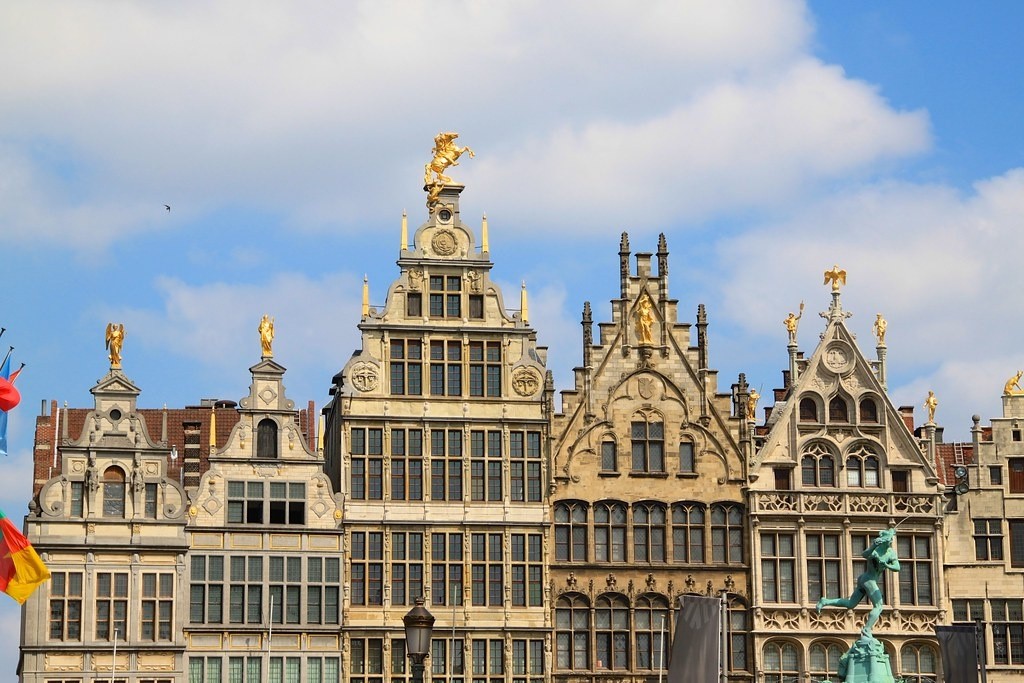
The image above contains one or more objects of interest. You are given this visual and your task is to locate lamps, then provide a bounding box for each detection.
[955,467,969,494]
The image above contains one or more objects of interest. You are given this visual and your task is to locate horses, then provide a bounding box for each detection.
[426,132,475,181]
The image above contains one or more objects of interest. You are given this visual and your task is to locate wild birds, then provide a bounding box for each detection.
[165,205,171,212]
[824,265,847,285]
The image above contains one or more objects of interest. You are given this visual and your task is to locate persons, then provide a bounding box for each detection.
[258,314,276,354]
[783,311,802,342]
[923,391,939,422]
[425,133,476,212]
[746,389,761,416]
[105,323,126,365]
[815,526,901,653]
[824,265,848,291]
[636,295,656,346]
[872,312,888,345]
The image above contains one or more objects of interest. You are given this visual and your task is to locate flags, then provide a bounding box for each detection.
[0,332,51,606]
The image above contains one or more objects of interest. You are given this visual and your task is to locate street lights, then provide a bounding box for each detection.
[402,596,436,683]
